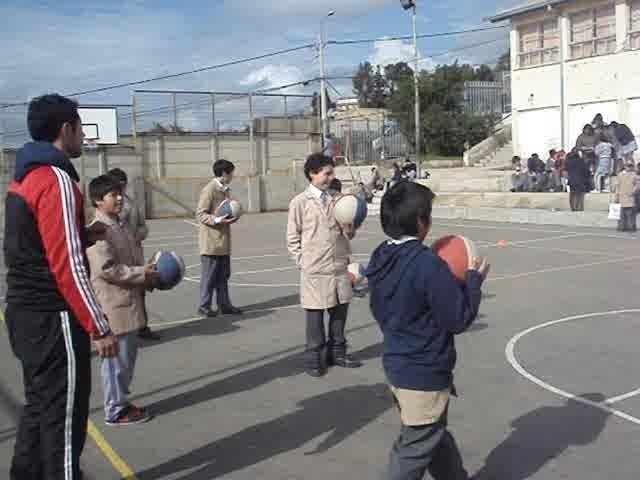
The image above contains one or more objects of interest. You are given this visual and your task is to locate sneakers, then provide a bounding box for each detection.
[104,404,154,427]
[136,327,161,340]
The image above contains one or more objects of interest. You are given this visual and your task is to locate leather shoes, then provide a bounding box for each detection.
[334,355,361,368]
[198,308,217,317]
[221,304,240,314]
[509,186,610,193]
[304,367,321,377]
[352,289,364,297]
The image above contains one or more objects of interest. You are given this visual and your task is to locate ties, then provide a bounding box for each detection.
[321,193,328,214]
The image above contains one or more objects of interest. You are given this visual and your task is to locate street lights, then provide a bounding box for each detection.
[318,10,337,162]
[401,0,421,179]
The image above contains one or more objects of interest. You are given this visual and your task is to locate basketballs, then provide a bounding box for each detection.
[430,234,477,285]
[334,195,369,227]
[346,262,364,286]
[217,200,241,221]
[153,249,184,291]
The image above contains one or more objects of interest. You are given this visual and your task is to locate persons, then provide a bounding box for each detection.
[87,170,157,426]
[509,112,639,231]
[365,182,490,480]
[106,167,162,341]
[194,157,242,316]
[287,151,361,377]
[320,133,430,204]
[4,92,121,480]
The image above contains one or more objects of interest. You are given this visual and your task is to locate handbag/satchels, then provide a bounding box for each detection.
[608,204,622,220]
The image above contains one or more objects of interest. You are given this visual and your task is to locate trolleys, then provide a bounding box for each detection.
[334,156,374,204]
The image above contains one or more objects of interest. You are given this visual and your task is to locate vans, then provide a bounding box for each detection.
[372,122,411,159]
